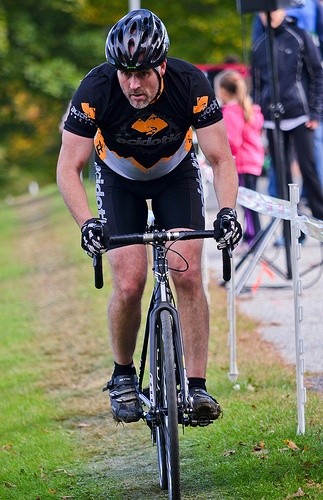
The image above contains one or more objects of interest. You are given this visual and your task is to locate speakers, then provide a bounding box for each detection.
[236,0,305,15]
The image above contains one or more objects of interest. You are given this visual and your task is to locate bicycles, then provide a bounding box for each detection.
[89,218,233,500]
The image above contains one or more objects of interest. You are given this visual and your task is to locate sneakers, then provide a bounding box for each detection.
[190,389,219,418]
[108,373,142,422]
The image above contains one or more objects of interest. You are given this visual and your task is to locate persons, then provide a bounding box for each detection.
[247,7,323,249]
[212,69,265,256]
[55,8,243,426]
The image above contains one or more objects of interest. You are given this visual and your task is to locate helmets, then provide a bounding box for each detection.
[105,9,169,71]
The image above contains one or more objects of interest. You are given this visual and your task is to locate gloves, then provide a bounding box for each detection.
[213,207,243,252]
[81,218,110,254]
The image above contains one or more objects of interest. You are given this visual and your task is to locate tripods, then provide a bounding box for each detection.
[220,12,323,289]
